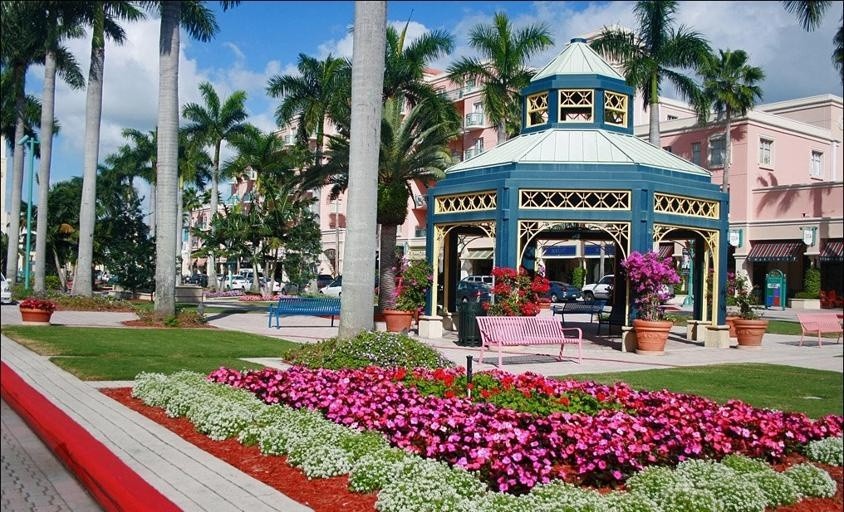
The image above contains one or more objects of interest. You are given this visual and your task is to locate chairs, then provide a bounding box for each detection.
[597,304,625,335]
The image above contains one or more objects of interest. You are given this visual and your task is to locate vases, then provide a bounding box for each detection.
[382,308,414,333]
[20,309,53,325]
[725,315,745,337]
[633,319,675,356]
[732,319,769,350]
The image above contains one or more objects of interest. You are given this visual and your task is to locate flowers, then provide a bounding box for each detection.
[620,251,680,321]
[482,264,551,317]
[725,271,768,320]
[386,251,434,315]
[19,298,57,312]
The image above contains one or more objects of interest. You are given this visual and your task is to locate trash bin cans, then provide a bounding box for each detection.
[457,302,487,347]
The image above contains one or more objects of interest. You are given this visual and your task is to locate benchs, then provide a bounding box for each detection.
[553,299,607,324]
[473,316,584,369]
[796,312,844,348]
[267,297,341,330]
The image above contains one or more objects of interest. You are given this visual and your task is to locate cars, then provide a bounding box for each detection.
[580,274,671,306]
[280,275,342,298]
[220,268,280,292]
[457,274,492,304]
[189,274,207,287]
[1,271,13,304]
[549,281,582,302]
